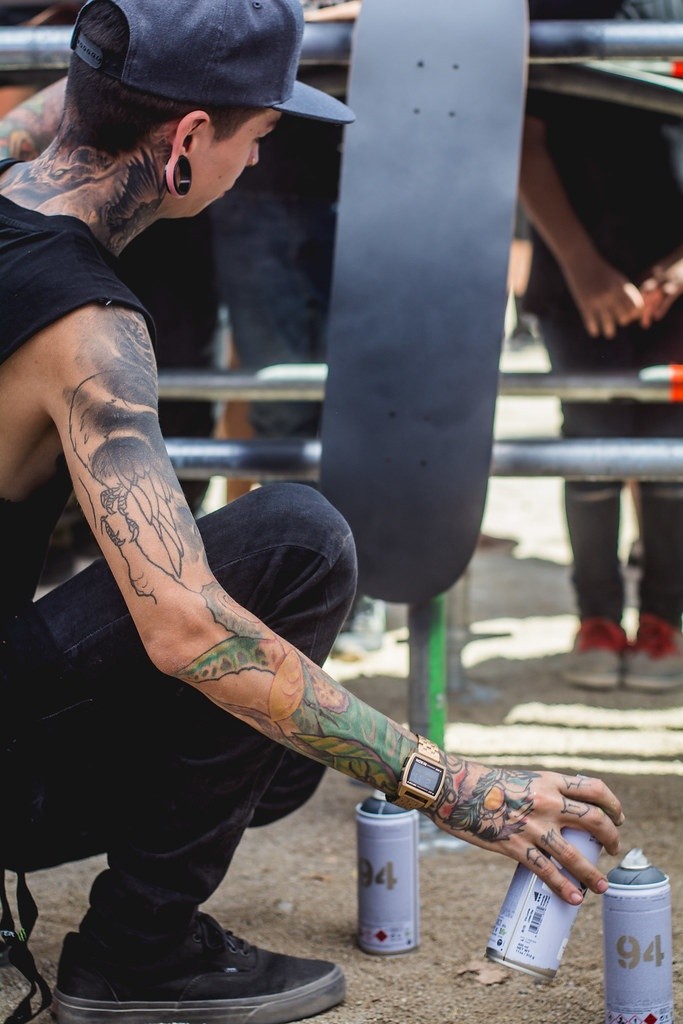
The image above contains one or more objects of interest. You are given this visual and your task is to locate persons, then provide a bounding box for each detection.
[519,0,682,690]
[1,0,629,1024]
[107,0,388,662]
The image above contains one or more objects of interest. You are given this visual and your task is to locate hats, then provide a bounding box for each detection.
[69,0,356,124]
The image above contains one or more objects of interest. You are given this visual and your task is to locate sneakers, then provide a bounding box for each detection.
[627,615,683,691]
[568,619,628,688]
[53,911,345,1024]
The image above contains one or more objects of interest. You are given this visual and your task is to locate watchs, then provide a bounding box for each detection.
[387,728,448,811]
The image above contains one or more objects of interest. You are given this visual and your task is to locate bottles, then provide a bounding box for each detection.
[484,826,604,983]
[604,849,675,1023]
[355,786,419,956]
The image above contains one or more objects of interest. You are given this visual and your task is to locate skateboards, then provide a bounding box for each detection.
[307,0,536,610]
